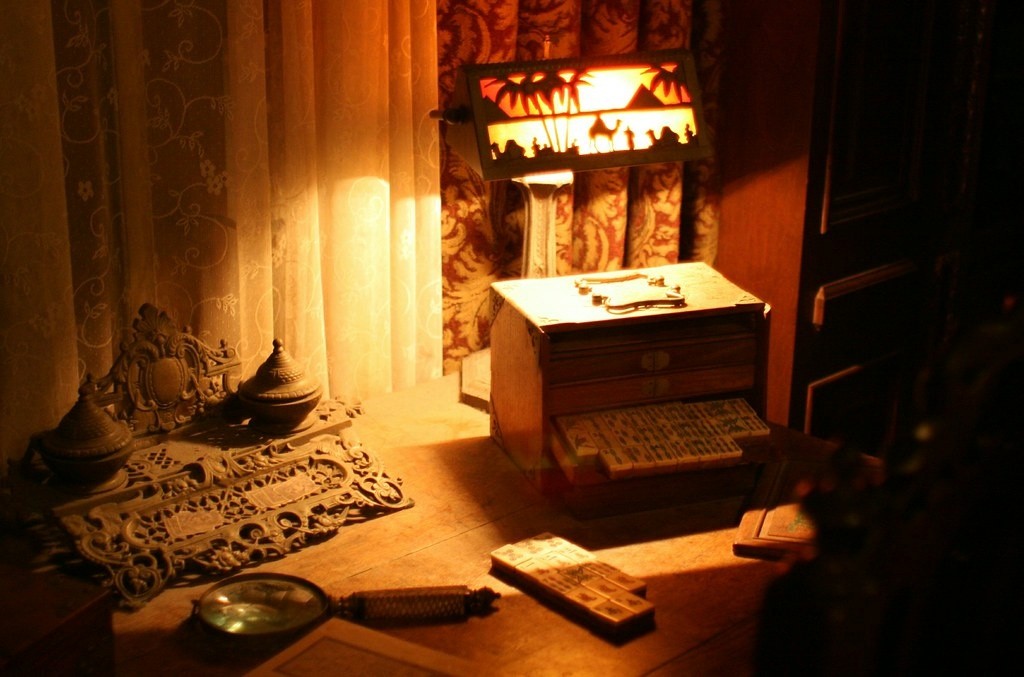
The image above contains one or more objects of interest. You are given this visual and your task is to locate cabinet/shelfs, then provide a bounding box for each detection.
[712,0,1001,450]
[487,258,774,512]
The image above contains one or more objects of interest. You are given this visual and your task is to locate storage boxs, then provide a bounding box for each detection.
[0,562,116,677]
